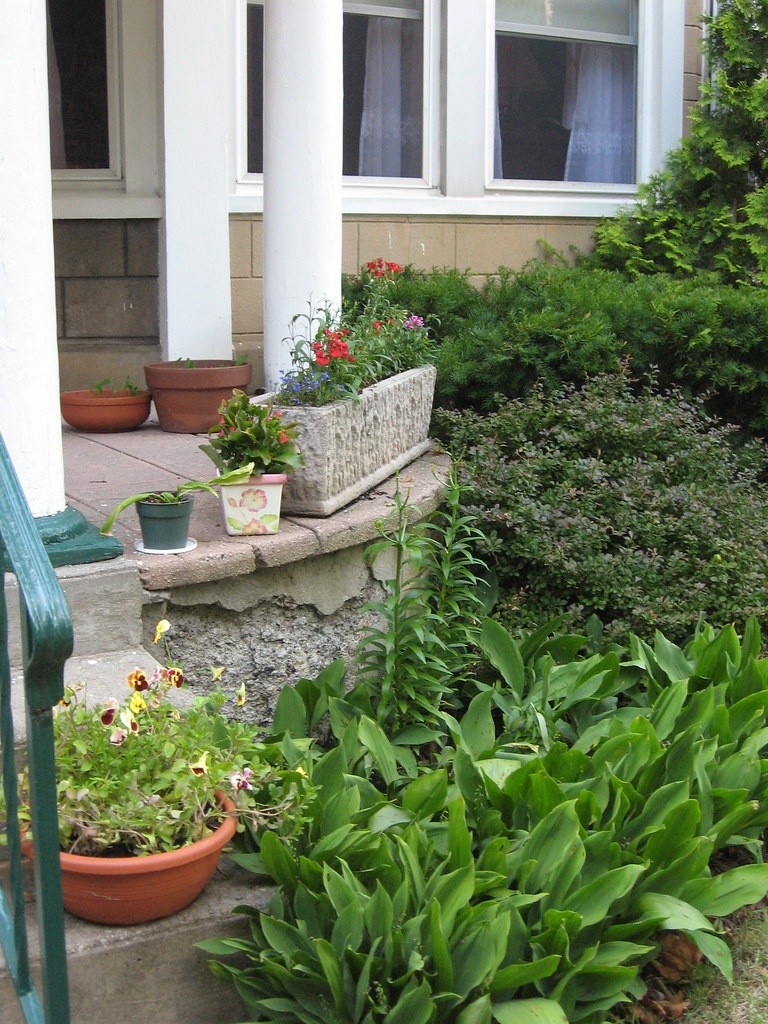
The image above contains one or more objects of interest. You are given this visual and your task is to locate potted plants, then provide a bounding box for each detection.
[59,374,153,434]
[99,461,257,551]
[144,353,253,434]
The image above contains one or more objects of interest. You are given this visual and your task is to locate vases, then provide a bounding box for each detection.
[248,364,438,517]
[216,467,289,536]
[20,788,238,925]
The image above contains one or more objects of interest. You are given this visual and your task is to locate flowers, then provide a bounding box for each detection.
[0,618,324,862]
[262,257,442,408]
[198,388,308,477]
